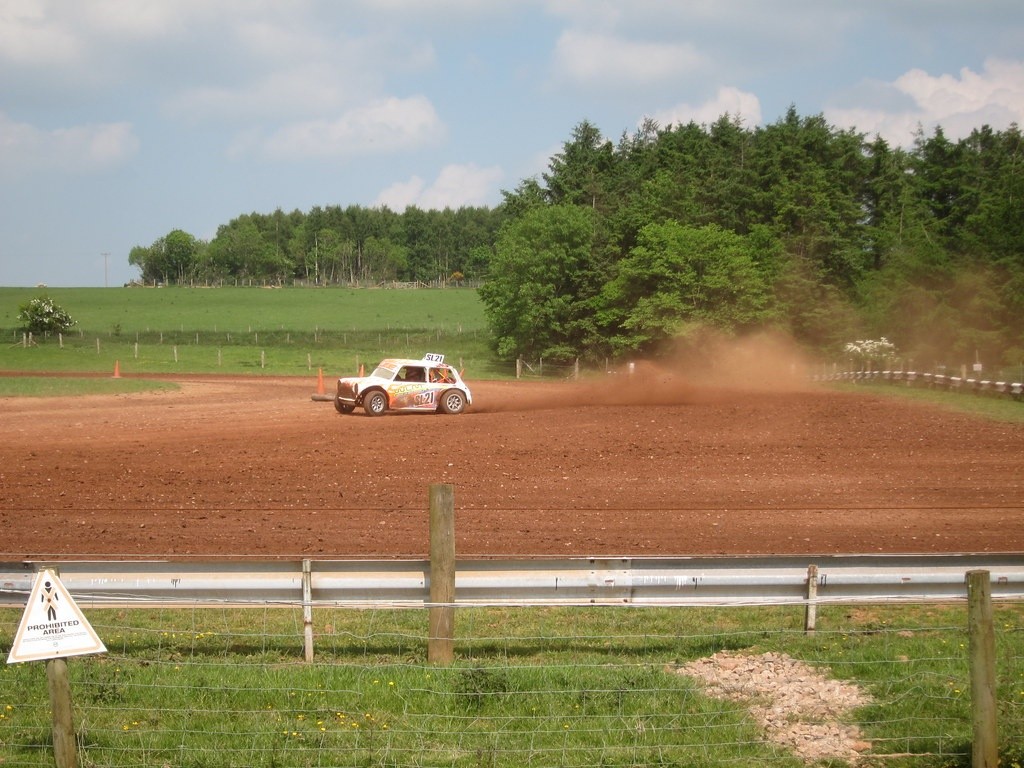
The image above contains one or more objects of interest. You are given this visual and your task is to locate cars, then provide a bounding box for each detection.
[333,352,473,417]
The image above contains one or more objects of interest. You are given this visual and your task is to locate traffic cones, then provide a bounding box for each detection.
[459,368,465,378]
[359,364,364,377]
[316,369,326,395]
[111,360,123,379]
